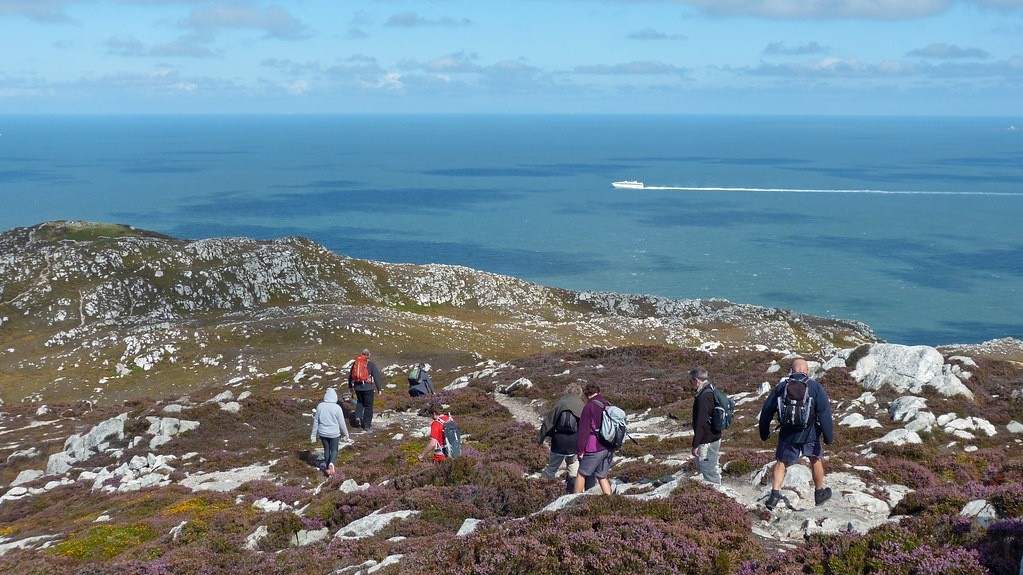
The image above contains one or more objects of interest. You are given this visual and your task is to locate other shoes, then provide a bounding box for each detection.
[326,470,330,475]
[364,427,370,430]
[355,418,362,428]
[329,462,334,474]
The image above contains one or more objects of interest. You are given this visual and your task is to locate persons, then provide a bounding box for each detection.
[417,403,455,461]
[536,384,585,483]
[408,362,434,397]
[689,368,721,484]
[348,348,381,430]
[759,359,833,508]
[311,388,349,474]
[573,384,614,494]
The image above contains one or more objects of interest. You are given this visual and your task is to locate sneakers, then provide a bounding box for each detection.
[815,487,832,506]
[765,493,781,509]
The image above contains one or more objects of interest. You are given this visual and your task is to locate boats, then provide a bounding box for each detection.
[611,180,645,190]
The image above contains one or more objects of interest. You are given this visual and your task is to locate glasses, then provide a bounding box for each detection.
[689,379,696,385]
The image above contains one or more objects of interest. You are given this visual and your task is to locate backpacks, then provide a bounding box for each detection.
[553,409,577,434]
[433,411,462,460]
[588,399,638,464]
[407,366,423,384]
[768,377,814,437]
[352,354,373,386]
[700,382,735,430]
